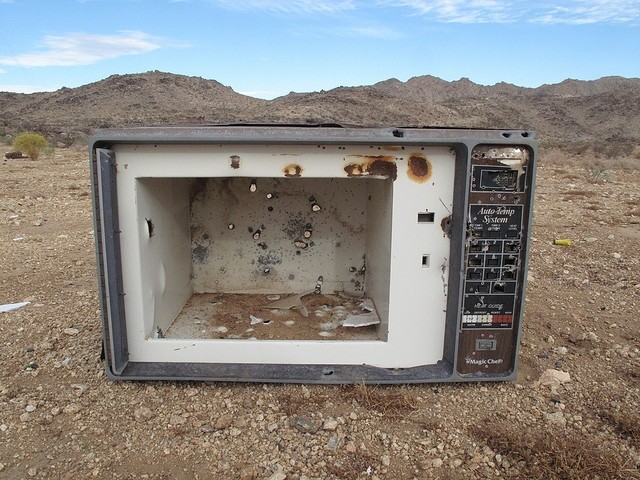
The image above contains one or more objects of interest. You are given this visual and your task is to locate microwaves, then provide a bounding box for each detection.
[91,125,535,383]
